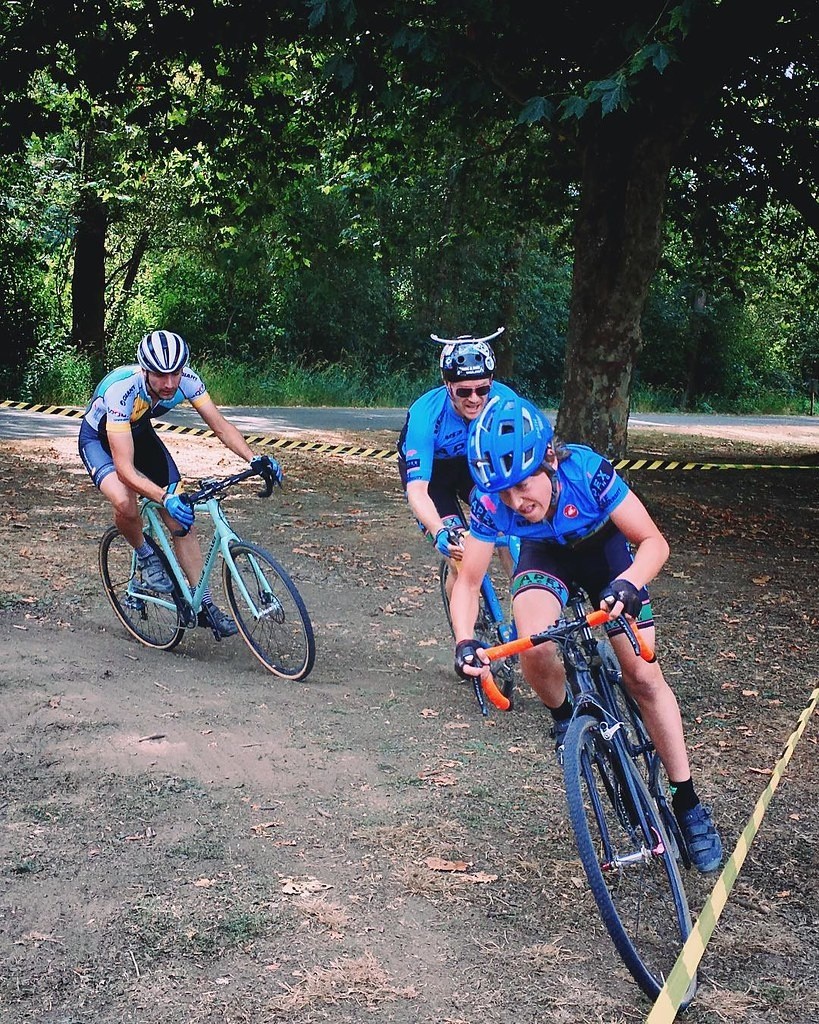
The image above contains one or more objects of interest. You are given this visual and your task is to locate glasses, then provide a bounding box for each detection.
[449,382,491,399]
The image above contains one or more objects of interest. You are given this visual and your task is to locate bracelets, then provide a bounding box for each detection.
[161,492,169,506]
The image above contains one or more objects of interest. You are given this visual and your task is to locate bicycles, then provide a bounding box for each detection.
[471,576,698,1015]
[97,455,316,682]
[438,528,522,699]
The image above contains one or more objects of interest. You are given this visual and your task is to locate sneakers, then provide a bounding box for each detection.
[673,804,723,872]
[201,605,240,635]
[555,719,572,766]
[136,552,174,593]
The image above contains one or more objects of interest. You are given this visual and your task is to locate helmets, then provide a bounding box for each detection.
[467,393,553,493]
[439,334,498,381]
[137,331,189,375]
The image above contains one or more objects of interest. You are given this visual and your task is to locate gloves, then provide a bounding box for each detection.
[250,455,282,485]
[161,493,194,530]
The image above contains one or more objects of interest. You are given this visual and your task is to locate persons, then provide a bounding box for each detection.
[449,393,723,874]
[76,330,285,637]
[395,336,521,625]
